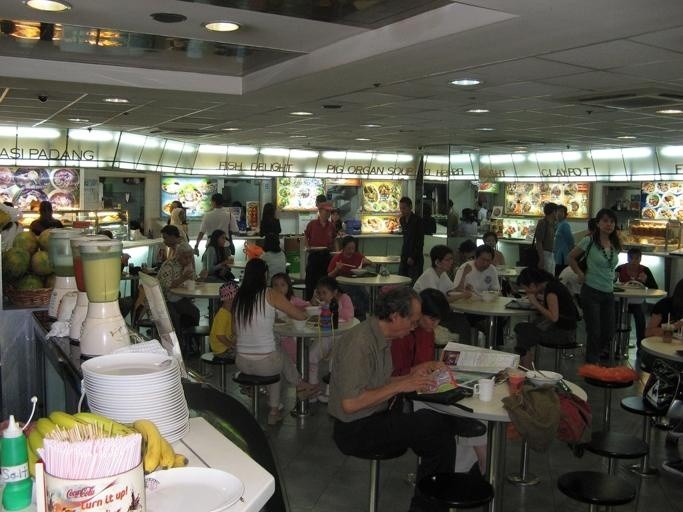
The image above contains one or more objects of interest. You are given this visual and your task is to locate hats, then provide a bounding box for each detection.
[218,280,240,301]
[318,202,337,212]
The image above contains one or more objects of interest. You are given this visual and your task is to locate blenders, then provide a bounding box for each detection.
[46,227,132,358]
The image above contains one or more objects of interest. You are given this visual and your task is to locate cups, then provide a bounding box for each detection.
[184,280,195,291]
[509,373,524,396]
[227,255,234,264]
[662,322,675,343]
[475,379,495,402]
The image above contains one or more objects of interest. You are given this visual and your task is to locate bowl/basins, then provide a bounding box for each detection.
[518,298,533,307]
[350,269,368,276]
[386,254,400,262]
[496,264,528,273]
[525,370,563,387]
[623,242,679,252]
[447,289,498,302]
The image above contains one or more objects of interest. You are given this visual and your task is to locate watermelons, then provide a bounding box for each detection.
[2,230,55,291]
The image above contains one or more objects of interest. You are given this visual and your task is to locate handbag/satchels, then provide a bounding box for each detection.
[533,313,555,333]
[577,233,595,275]
[641,356,680,417]
[524,217,547,268]
[399,382,476,414]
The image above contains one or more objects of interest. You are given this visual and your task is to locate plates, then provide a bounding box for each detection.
[162,181,218,216]
[0,166,80,210]
[74,215,122,224]
[504,183,581,238]
[364,186,403,231]
[642,182,683,223]
[280,178,326,211]
[614,284,641,289]
[82,354,190,446]
[308,247,342,256]
[144,468,244,512]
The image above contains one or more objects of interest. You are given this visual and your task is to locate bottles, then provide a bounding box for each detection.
[130,226,140,240]
[380,264,390,277]
[319,296,339,331]
[0,396,38,512]
[644,287,648,292]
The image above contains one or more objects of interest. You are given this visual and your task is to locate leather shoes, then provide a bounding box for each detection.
[455,416,486,437]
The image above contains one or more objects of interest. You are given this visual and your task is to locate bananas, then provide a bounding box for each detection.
[26,411,188,478]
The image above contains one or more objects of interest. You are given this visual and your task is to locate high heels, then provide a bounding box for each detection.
[267,410,284,425]
[296,382,320,402]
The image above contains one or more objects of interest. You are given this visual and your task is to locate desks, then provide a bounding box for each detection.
[133,234,683,512]
